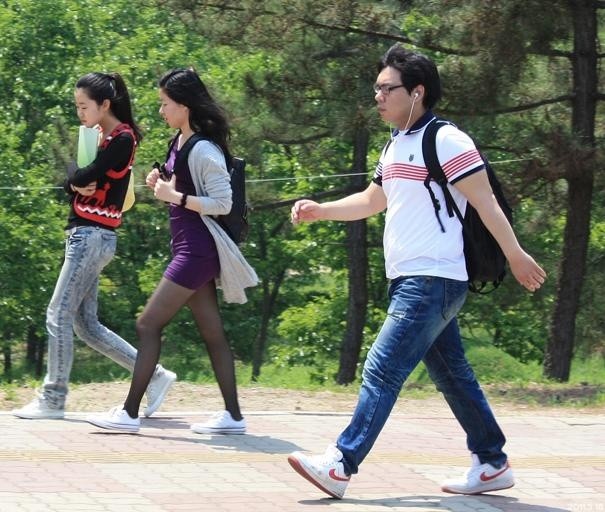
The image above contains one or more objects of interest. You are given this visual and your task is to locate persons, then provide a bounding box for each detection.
[10,71,179,420]
[281,40,548,502]
[82,69,258,436]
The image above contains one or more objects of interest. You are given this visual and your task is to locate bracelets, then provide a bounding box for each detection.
[178,192,187,209]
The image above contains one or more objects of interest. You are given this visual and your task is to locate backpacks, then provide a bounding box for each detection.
[461,155,515,284]
[213,157,247,244]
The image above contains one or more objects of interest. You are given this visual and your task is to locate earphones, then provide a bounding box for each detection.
[414,93,418,99]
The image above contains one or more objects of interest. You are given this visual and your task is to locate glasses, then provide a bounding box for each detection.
[372,82,410,95]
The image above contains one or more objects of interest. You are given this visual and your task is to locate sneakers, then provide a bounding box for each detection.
[86,404,141,434]
[190,409,252,435]
[287,442,349,503]
[143,363,177,417]
[10,392,65,419]
[440,453,516,493]
